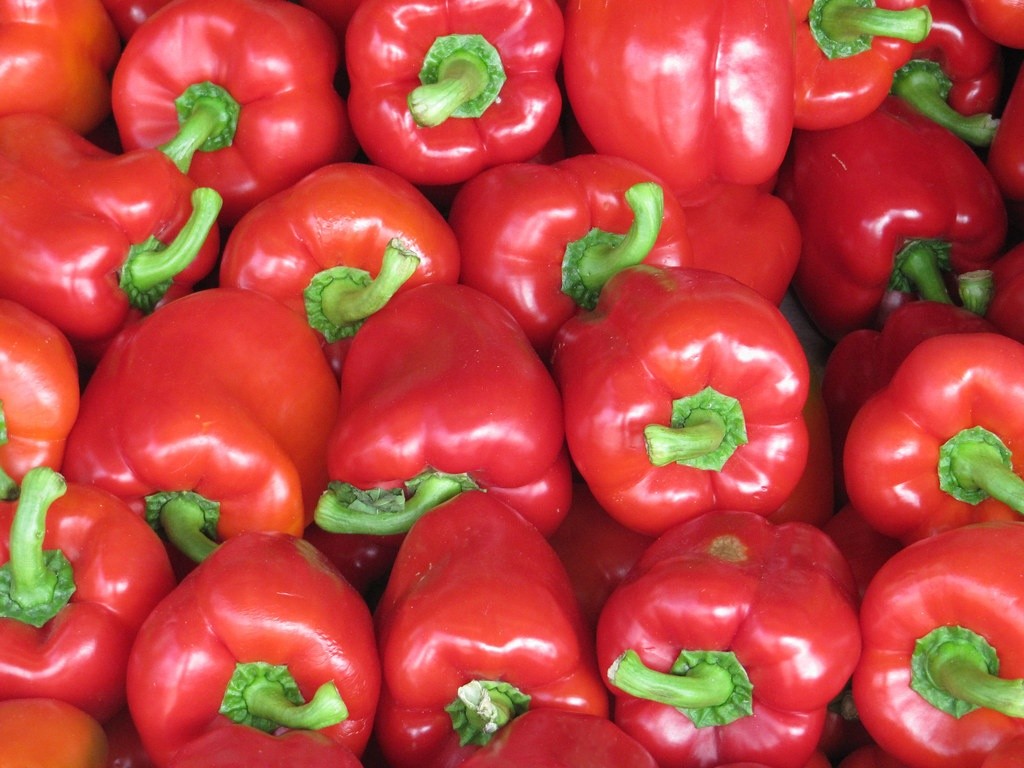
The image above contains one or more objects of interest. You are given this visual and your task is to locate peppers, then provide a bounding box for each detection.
[0,0,1024,768]
